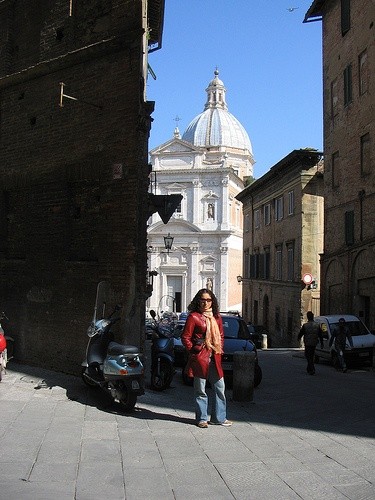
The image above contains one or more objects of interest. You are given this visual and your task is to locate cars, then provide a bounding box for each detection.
[311,314,375,370]
[246,323,272,349]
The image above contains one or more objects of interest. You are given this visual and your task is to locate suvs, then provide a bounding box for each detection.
[146,311,262,391]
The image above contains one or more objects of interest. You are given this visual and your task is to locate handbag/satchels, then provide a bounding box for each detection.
[184,362,194,378]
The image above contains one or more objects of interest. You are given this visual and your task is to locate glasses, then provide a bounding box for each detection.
[200,299,213,302]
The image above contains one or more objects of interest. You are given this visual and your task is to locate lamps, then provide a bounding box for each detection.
[237,274,245,285]
[146,231,174,256]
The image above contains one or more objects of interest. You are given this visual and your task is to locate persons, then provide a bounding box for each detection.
[182,289,232,428]
[297,312,324,375]
[207,279,213,290]
[329,318,354,372]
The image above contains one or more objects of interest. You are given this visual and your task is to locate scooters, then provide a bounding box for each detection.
[80,281,146,411]
[147,295,184,392]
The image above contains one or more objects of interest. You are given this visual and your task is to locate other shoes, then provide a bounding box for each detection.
[307,367,315,375]
[209,420,233,426]
[198,422,208,428]
